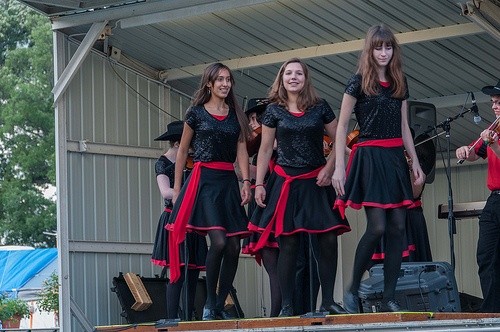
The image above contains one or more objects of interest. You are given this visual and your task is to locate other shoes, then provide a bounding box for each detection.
[278,306,294,318]
[380,299,408,312]
[344,292,361,314]
[215,308,237,319]
[320,304,347,314]
[203,306,213,321]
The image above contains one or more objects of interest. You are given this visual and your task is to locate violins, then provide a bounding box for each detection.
[247,124,263,157]
[487,116,500,145]
[346,130,361,150]
[185,149,196,171]
[323,135,335,156]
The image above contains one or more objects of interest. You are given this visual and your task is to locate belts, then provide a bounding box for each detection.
[492,191,500,194]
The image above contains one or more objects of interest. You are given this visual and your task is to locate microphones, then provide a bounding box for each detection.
[470,92,481,124]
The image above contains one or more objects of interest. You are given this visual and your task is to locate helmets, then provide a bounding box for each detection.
[243,97,272,115]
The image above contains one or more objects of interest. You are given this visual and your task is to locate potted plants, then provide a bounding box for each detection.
[0,295,33,328]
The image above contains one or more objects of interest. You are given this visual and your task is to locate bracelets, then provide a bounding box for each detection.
[241,180,251,183]
[255,185,264,187]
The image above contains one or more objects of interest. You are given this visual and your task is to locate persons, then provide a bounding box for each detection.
[152,98,434,319]
[246,57,351,318]
[456,79,500,313]
[332,23,425,314]
[165,61,251,321]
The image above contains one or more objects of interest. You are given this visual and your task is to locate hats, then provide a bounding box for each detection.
[154,121,184,143]
[481,81,500,96]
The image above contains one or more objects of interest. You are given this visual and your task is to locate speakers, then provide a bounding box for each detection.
[357,261,460,313]
[405,101,437,182]
